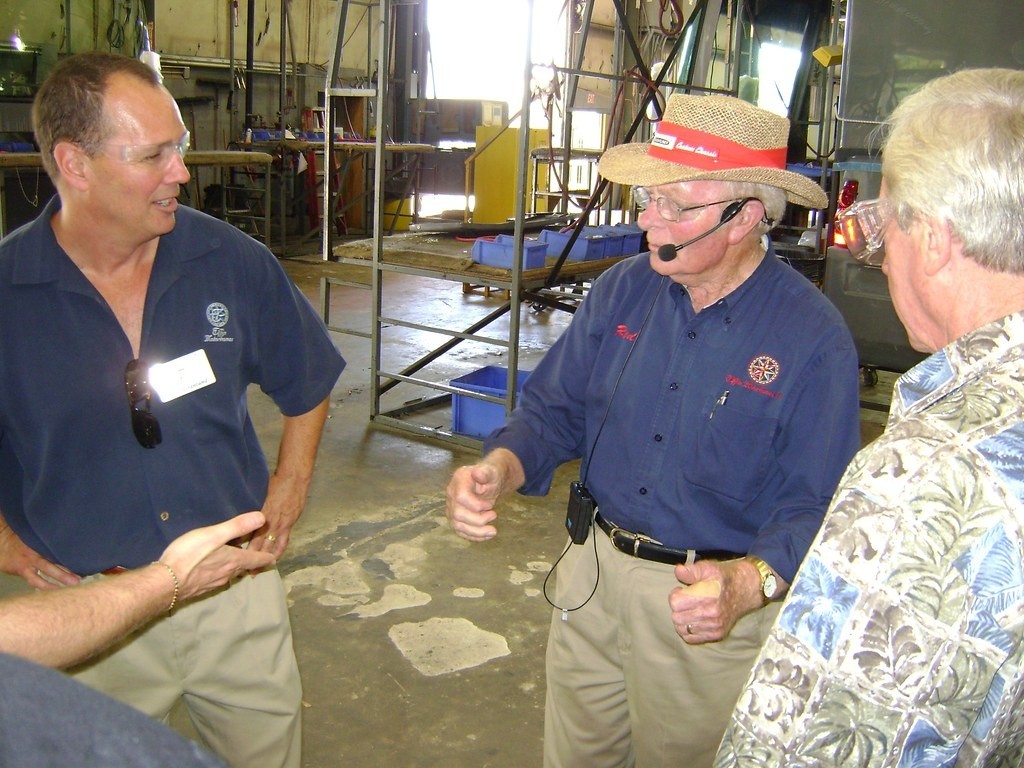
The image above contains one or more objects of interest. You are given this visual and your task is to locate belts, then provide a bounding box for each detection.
[100,563,128,579]
[595,510,747,566]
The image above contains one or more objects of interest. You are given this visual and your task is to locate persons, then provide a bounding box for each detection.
[1,51,347,768]
[444,96,862,768]
[710,67,1024,768]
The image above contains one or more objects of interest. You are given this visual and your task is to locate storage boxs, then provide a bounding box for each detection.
[537,230,604,261]
[470,235,549,270]
[597,224,642,255]
[584,226,624,259]
[449,364,536,438]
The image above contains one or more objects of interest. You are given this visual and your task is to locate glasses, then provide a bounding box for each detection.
[839,198,959,268]
[631,186,751,221]
[125,359,162,448]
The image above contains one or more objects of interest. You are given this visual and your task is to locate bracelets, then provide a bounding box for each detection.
[152,561,177,613]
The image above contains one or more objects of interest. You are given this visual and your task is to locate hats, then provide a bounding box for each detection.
[598,93,828,209]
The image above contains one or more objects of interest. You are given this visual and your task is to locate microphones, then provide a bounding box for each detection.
[657,198,750,262]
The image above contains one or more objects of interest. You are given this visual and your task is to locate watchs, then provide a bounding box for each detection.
[744,557,777,608]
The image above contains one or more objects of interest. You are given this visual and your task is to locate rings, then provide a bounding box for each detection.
[687,624,693,635]
[265,535,276,543]
